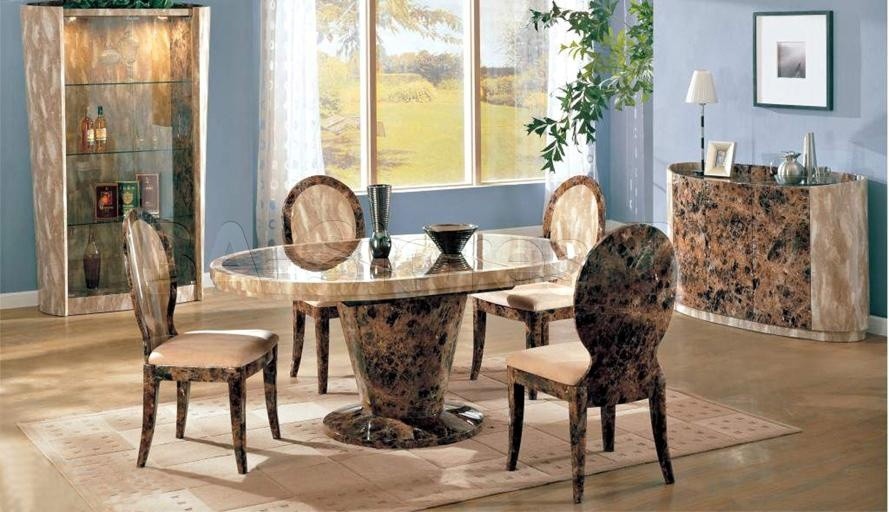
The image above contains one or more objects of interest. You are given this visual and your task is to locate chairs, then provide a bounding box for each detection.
[468,174,608,400]
[505,220,677,505]
[119,206,282,475]
[283,175,366,395]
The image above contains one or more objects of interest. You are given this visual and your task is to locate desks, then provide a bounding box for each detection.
[664,160,871,344]
[208,229,588,449]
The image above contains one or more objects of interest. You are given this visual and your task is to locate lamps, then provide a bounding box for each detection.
[684,69,719,176]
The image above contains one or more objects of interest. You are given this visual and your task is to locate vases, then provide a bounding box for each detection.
[776,151,807,185]
[804,132,817,176]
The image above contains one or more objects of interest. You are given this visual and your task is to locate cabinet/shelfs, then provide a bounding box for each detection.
[18,0,211,317]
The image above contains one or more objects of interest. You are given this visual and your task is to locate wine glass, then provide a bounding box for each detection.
[100,47,122,83]
[119,43,138,84]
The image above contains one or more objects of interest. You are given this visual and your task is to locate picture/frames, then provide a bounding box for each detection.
[752,8,834,111]
[703,138,736,178]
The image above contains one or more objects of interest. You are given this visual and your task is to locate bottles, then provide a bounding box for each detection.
[78,106,95,154]
[800,130,818,183]
[92,106,108,153]
[81,230,101,290]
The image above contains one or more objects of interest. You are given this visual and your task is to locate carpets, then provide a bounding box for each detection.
[14,349,802,512]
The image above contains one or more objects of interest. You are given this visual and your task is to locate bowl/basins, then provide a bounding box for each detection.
[423,221,478,255]
[424,253,475,275]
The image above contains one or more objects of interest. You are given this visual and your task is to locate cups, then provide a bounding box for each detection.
[768,151,806,185]
[368,254,392,280]
[807,166,834,185]
